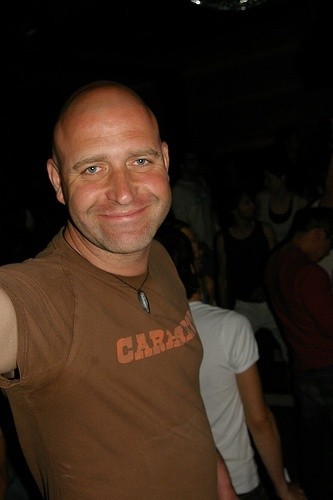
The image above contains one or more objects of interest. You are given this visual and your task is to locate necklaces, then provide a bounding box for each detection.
[61,225,150,315]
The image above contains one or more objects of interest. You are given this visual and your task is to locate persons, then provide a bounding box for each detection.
[153,225,305,500]
[257,239,333,344]
[250,155,310,244]
[169,145,223,307]
[165,216,221,307]
[276,205,333,417]
[213,183,275,388]
[1,79,236,500]
[307,163,333,208]
